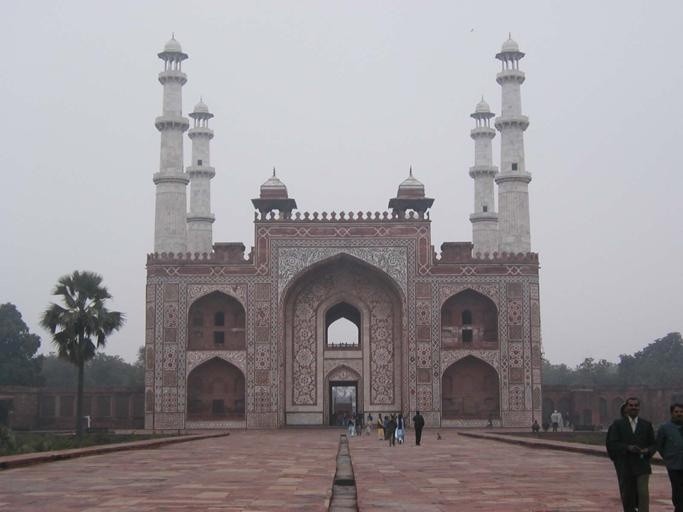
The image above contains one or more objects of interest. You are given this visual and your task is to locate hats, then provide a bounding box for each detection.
[631,418,636,434]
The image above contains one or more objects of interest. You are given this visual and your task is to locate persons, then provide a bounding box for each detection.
[530,409,576,432]
[606,404,642,508]
[655,402,683,512]
[412,411,425,446]
[607,397,656,512]
[340,411,405,447]
